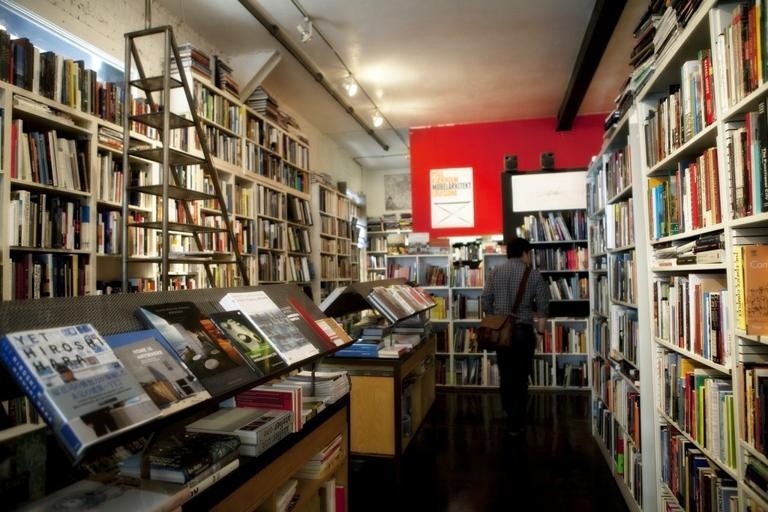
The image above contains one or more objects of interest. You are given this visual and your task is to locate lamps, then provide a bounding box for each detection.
[295,15,315,47]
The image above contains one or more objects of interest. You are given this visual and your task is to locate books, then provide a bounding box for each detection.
[156,44,316,290]
[0,33,155,302]
[588,0,767,510]
[0,291,351,512]
[450,208,592,389]
[319,187,360,299]
[334,237,450,387]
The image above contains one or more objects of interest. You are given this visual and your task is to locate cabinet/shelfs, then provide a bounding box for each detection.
[585,0,768,510]
[366,210,592,389]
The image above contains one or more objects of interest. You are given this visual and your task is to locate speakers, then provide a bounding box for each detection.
[541,152,554,169]
[504,155,517,171]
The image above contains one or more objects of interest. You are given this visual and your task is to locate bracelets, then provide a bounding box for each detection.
[536,331,544,335]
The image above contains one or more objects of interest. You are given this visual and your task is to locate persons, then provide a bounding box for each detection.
[480,238,550,437]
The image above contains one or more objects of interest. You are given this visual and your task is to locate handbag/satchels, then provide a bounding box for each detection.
[477,314,516,350]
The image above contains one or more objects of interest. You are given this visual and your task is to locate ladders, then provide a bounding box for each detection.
[123,25,250,292]
